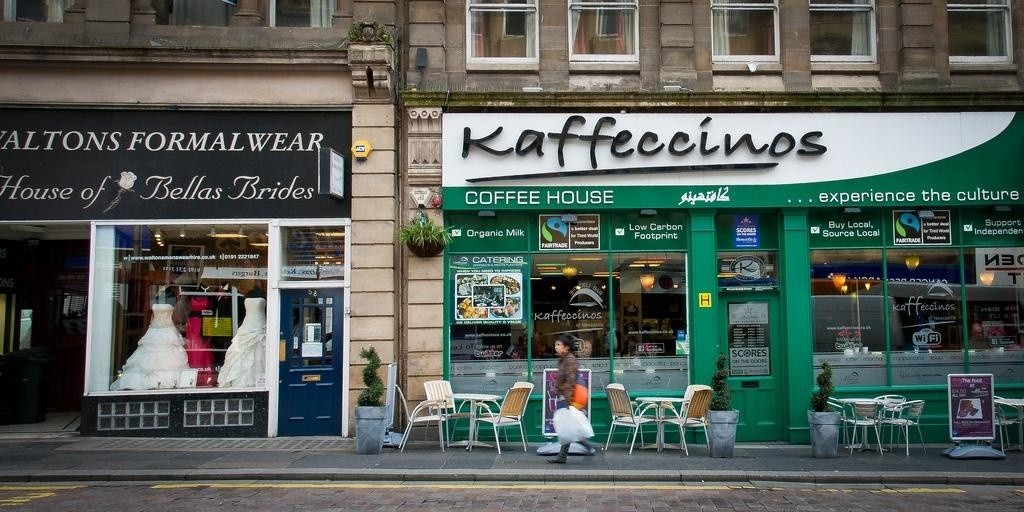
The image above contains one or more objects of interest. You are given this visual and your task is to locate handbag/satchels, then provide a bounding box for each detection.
[572,384,588,409]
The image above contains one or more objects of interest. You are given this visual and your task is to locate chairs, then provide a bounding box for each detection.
[396,379,535,454]
[604,383,714,456]
[827,394,925,457]
[994,395,1024,455]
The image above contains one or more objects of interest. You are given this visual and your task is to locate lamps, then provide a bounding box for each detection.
[904,255,920,270]
[639,268,656,290]
[979,270,995,286]
[830,272,871,294]
[154,231,185,247]
[248,243,268,247]
[206,227,249,242]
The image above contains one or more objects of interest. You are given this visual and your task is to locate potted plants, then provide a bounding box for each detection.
[395,218,455,258]
[705,353,740,459]
[807,361,841,459]
[354,344,390,456]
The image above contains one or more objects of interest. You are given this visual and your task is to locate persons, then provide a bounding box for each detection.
[966,320,994,349]
[546,334,596,464]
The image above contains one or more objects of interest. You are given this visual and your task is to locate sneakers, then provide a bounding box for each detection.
[547,456,566,463]
